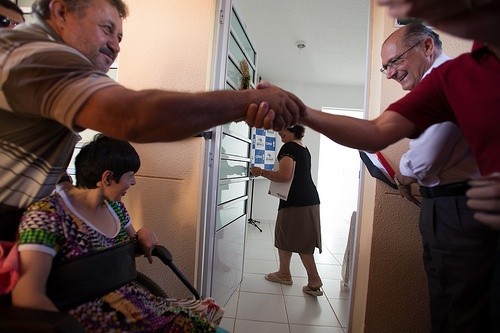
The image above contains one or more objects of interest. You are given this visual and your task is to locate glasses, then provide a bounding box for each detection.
[380,39,423,73]
[0,15,19,28]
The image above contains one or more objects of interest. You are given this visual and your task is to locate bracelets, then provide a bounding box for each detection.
[393,176,409,188]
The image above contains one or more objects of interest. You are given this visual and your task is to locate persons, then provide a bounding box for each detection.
[247,1,500,333]
[0,0,25,28]
[380,20,500,333]
[12,134,229,333]
[250,126,323,297]
[0,0,308,333]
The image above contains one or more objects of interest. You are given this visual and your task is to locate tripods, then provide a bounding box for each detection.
[248,178,262,232]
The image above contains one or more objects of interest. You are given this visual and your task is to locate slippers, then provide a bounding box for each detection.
[265,273,293,285]
[303,285,323,296]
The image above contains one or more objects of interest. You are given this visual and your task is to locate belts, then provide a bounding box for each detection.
[418,180,469,200]
